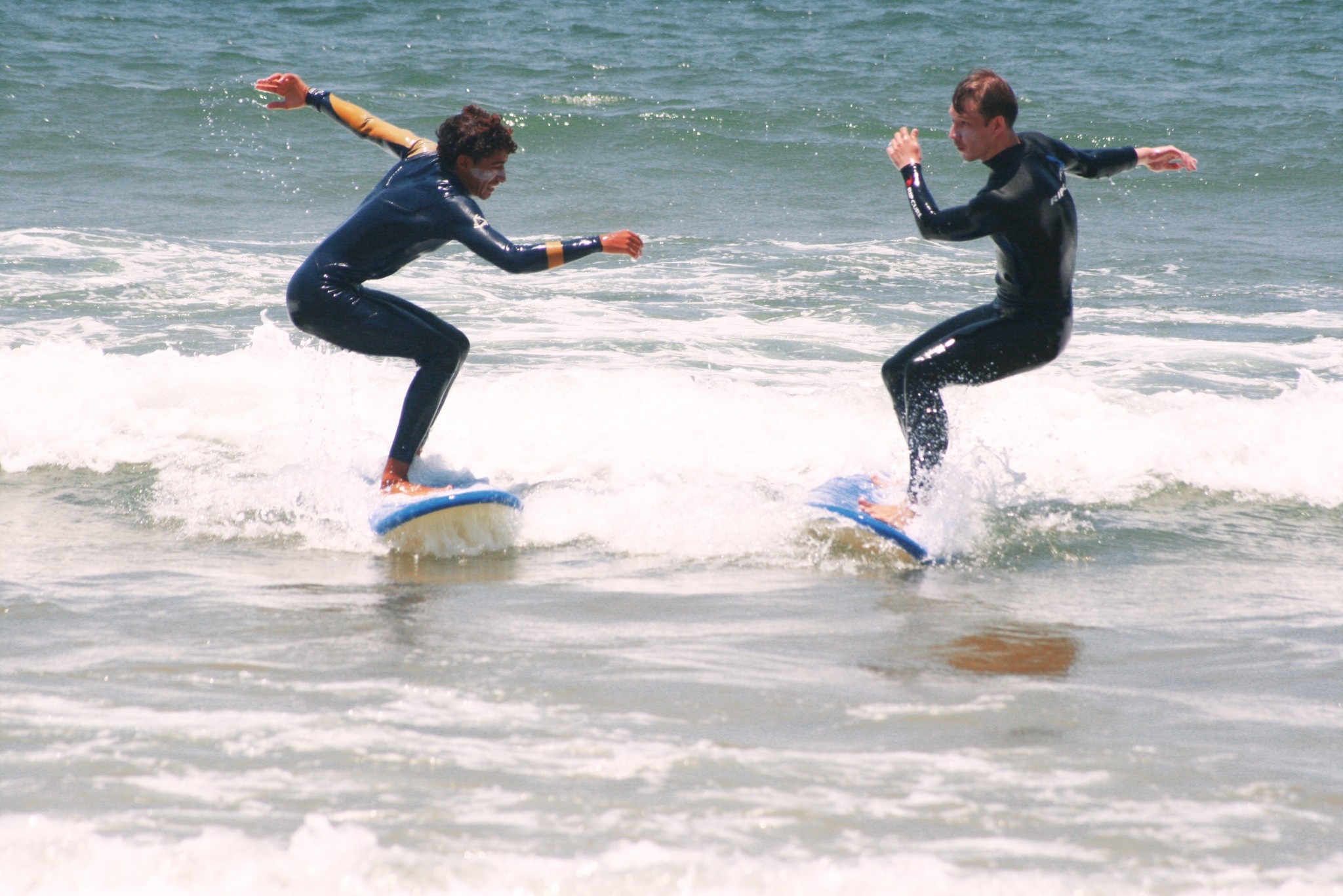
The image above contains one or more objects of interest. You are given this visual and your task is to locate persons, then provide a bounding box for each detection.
[254,73,644,500]
[857,69,1198,531]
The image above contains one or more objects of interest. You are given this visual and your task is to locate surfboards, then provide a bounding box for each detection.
[374,493,520,542]
[804,474,931,568]
